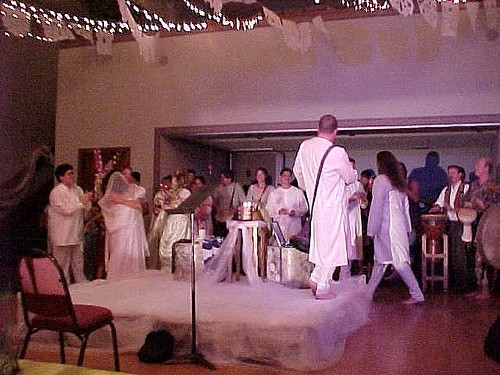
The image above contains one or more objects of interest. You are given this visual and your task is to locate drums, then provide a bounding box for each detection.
[420,212,446,264]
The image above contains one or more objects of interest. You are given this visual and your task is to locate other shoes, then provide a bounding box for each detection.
[308,278,337,300]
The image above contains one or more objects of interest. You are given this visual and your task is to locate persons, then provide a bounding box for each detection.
[83,171,148,234]
[461,156,500,301]
[293,114,358,301]
[97,170,149,281]
[190,176,214,238]
[246,167,274,201]
[48,162,95,288]
[332,158,368,282]
[265,168,317,246]
[361,152,425,304]
[183,169,196,188]
[360,169,376,200]
[395,160,421,243]
[428,166,477,298]
[155,174,191,270]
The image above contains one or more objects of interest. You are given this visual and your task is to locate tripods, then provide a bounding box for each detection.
[157,215,215,370]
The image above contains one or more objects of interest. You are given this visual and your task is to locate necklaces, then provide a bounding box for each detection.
[214,168,245,237]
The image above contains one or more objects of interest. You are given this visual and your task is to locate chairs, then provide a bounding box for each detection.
[17,247,120,371]
